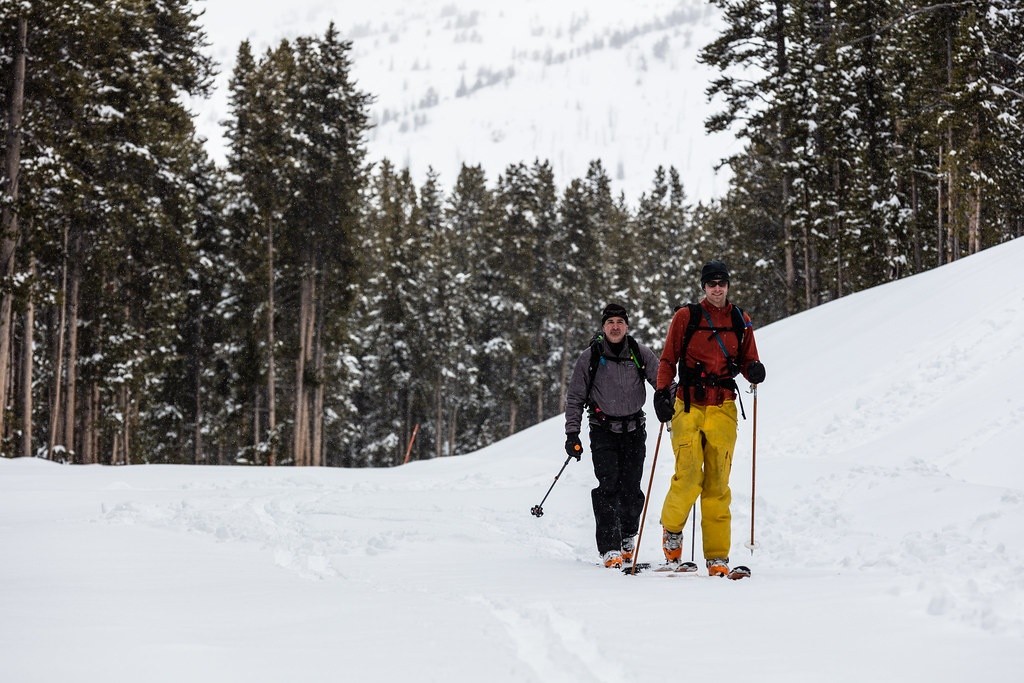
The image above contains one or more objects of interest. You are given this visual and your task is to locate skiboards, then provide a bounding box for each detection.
[595,562,651,574]
[659,562,751,580]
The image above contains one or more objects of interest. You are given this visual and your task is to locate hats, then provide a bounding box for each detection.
[700,260,730,289]
[601,304,628,327]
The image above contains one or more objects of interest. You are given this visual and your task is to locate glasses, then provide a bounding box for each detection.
[706,280,728,287]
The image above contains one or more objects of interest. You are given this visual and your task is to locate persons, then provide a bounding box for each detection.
[563,304,678,568]
[654,263,764,574]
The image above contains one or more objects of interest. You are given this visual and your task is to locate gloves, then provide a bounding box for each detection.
[653,387,676,423]
[565,436,583,458]
[747,361,766,384]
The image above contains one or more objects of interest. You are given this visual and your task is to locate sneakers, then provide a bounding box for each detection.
[662,526,683,563]
[621,536,635,559]
[705,557,729,576]
[603,550,622,568]
